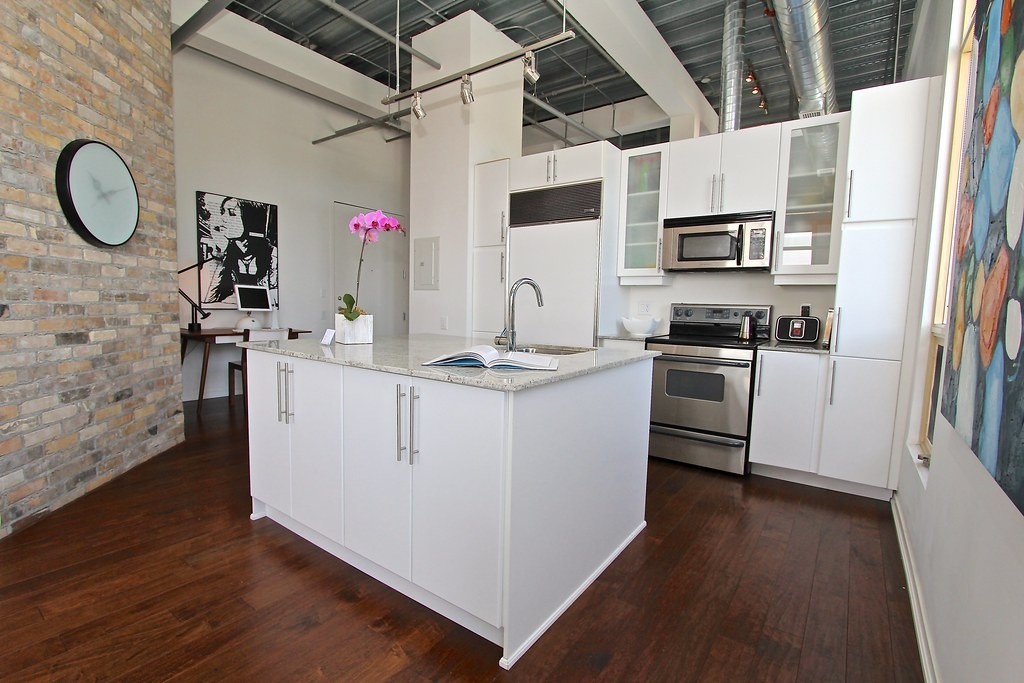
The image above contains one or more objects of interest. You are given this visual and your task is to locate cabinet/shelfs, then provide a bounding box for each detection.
[749,350,828,487]
[472,158,509,336]
[771,111,852,286]
[247,350,344,563]
[666,122,782,219]
[344,358,653,670]
[815,76,931,501]
[510,141,622,193]
[616,142,674,285]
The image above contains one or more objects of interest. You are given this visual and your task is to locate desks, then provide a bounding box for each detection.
[180,329,312,416]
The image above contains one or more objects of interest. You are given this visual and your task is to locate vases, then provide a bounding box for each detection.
[335,314,374,345]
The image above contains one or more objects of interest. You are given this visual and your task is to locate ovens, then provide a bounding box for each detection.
[645,343,757,476]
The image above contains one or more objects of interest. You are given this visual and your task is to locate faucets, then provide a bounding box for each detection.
[493,277,544,351]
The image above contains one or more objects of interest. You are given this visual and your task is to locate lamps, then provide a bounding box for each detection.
[746,70,754,83]
[752,84,759,95]
[177,252,225,331]
[381,0,576,120]
[759,100,765,108]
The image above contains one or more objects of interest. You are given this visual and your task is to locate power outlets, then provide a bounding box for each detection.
[638,303,652,315]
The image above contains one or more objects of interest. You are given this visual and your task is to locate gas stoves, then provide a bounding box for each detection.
[645,303,772,350]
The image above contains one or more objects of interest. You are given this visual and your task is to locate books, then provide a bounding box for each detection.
[423,345,558,372]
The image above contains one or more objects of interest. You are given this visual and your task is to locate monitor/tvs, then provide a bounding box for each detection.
[234,284,272,330]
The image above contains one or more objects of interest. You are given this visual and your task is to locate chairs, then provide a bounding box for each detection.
[228,328,292,415]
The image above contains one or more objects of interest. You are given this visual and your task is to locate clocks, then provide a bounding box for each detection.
[55,139,140,249]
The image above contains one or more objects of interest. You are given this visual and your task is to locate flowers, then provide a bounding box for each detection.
[338,209,407,321]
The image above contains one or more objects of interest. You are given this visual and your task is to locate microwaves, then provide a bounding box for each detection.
[662,211,775,271]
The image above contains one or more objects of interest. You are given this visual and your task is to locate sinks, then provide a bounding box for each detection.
[504,346,587,355]
